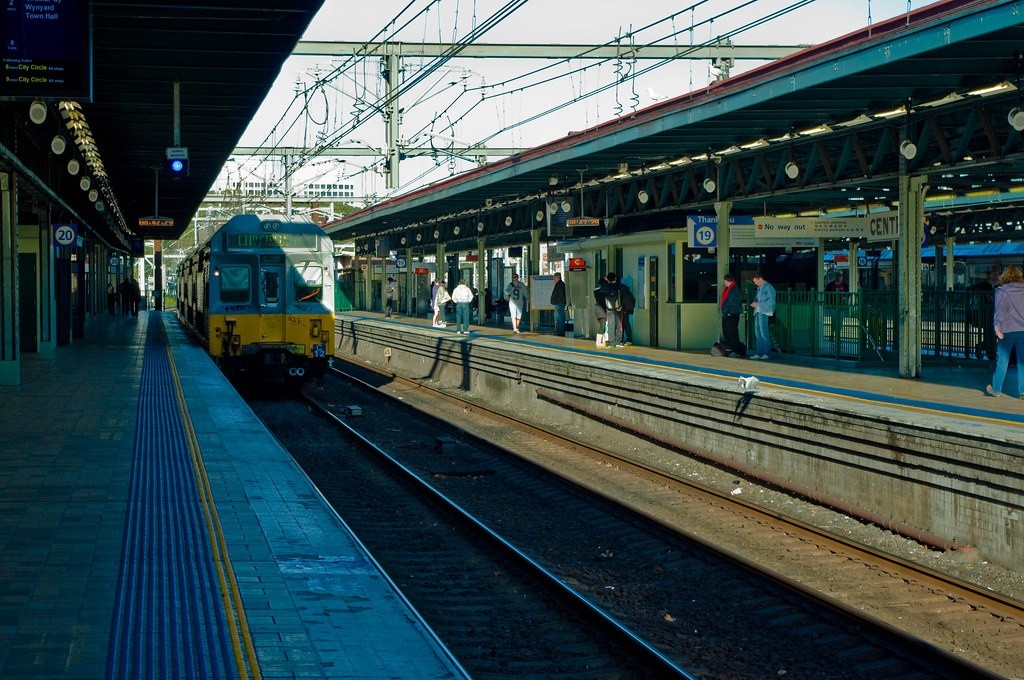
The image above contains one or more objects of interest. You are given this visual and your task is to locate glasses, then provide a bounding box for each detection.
[513,276,518,278]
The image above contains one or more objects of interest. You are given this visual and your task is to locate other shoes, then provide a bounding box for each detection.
[760,355,769,359]
[616,342,625,348]
[986,384,1001,397]
[623,342,632,346]
[464,331,470,335]
[749,354,760,359]
[432,324,439,327]
[457,332,461,334]
[728,352,746,358]
[513,329,520,335]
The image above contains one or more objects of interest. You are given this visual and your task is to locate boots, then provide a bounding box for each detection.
[596,333,607,347]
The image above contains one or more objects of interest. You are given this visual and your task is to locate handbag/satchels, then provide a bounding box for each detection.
[439,289,451,303]
[504,282,514,302]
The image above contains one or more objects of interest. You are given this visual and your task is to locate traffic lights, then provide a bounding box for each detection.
[167,158,189,177]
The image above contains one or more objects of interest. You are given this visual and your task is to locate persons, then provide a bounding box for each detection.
[503,274,530,334]
[106,278,142,319]
[969,264,1024,400]
[385,277,396,319]
[720,275,743,357]
[452,278,474,335]
[749,272,776,360]
[823,271,850,342]
[593,273,636,348]
[550,273,566,337]
[431,277,451,327]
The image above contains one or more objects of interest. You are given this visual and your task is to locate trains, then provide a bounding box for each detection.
[177,213,335,386]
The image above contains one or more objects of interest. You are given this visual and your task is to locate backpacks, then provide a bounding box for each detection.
[604,285,621,310]
